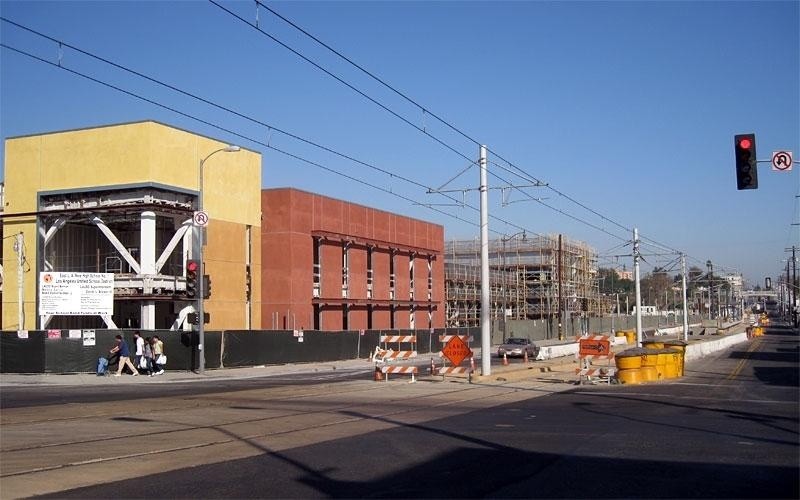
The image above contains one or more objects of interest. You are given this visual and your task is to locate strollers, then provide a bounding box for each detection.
[96,351,111,377]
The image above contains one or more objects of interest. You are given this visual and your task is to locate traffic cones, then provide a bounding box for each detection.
[502,354,509,366]
[524,350,528,363]
[375,355,383,380]
[470,357,476,370]
[430,357,435,376]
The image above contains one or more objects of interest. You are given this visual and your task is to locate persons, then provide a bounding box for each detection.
[142,337,156,377]
[152,335,165,375]
[133,331,151,376]
[109,334,139,377]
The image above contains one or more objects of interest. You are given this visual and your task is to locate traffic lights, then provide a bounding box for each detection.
[204,277,211,300]
[737,134,761,191]
[185,261,200,301]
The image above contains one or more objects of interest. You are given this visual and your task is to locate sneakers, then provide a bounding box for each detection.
[113,373,121,377]
[126,369,165,377]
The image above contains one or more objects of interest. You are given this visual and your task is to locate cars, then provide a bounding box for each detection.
[500,338,536,357]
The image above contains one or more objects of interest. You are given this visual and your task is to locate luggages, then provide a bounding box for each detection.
[96,356,111,376]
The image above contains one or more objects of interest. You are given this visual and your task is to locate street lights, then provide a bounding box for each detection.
[198,147,242,370]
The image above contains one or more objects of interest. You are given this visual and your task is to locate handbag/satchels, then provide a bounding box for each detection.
[140,356,154,369]
[156,354,166,365]
